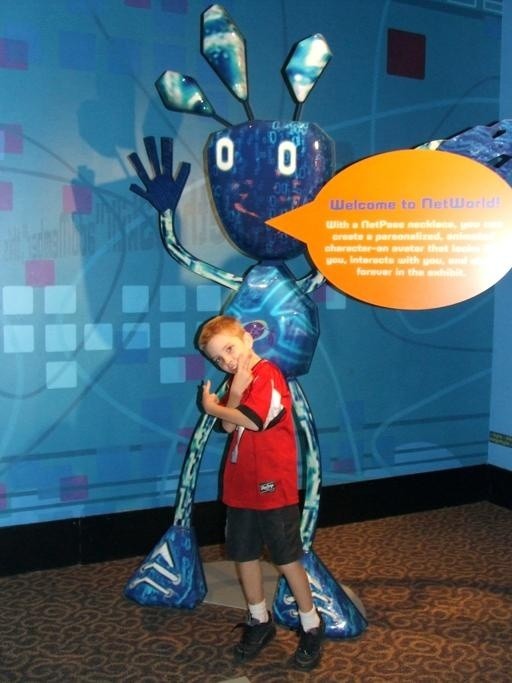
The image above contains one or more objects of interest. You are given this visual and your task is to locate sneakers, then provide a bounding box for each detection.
[294,609,323,669]
[235,611,277,662]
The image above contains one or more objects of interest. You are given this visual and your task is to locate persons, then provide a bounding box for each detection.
[196,315,325,669]
